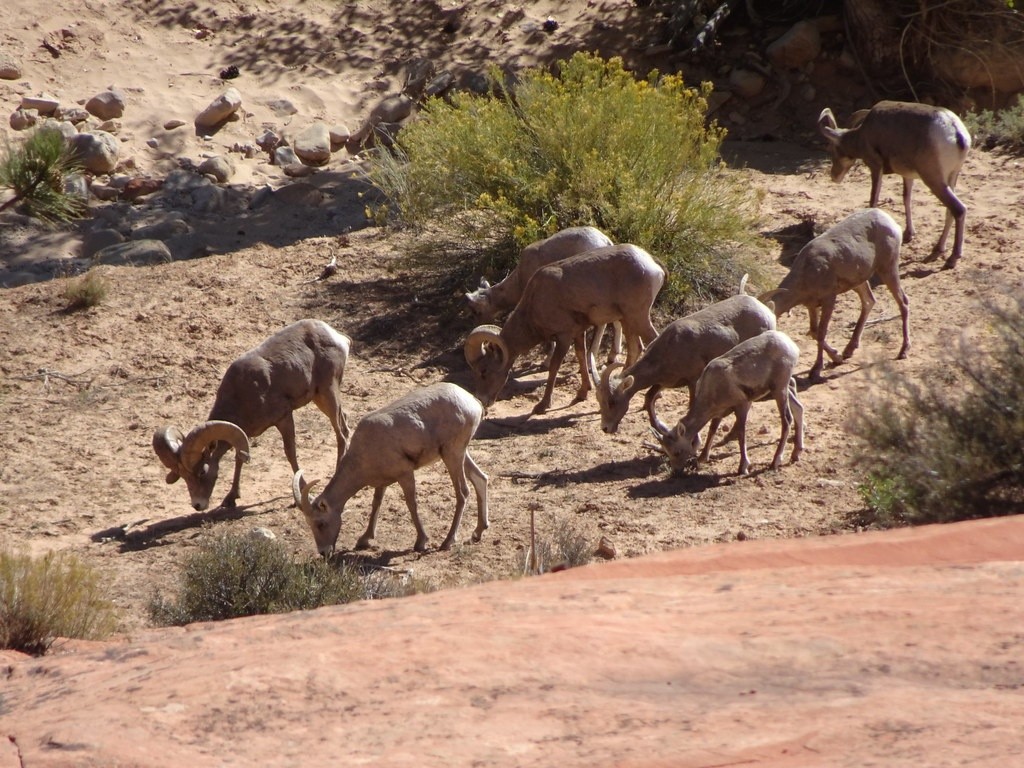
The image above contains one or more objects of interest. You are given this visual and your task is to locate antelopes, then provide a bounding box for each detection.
[817,100,972,270]
[151,318,489,562]
[462,207,912,478]
